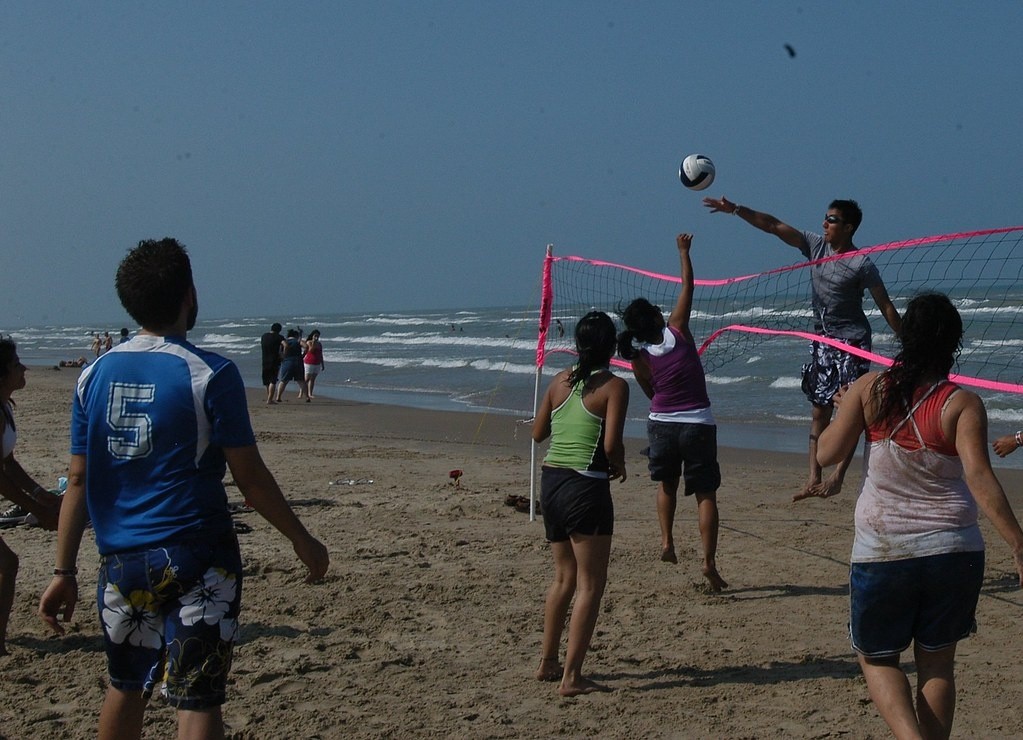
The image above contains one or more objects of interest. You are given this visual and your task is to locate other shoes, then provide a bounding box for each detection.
[515,496,541,514]
[505,494,518,506]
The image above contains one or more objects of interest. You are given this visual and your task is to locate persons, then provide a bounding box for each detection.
[816,290,1023,740]
[103,332,113,352]
[276,329,311,402]
[92,334,102,357]
[296,330,324,398]
[992,432,1023,458]
[452,323,455,331]
[617,233,728,589]
[0,332,62,656]
[38,239,327,740]
[120,328,130,343]
[261,323,287,405]
[532,310,630,696]
[556,319,564,337]
[702,195,903,501]
[60,357,89,368]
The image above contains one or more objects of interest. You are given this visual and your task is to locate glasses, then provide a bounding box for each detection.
[825,214,851,224]
[652,304,662,314]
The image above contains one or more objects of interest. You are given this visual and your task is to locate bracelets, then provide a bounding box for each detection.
[1014,431,1022,446]
[732,203,740,216]
[32,486,42,498]
[53,567,79,577]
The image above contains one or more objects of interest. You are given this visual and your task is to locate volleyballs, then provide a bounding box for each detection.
[678,153,716,192]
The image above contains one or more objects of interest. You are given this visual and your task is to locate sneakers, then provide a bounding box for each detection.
[0,505,27,522]
